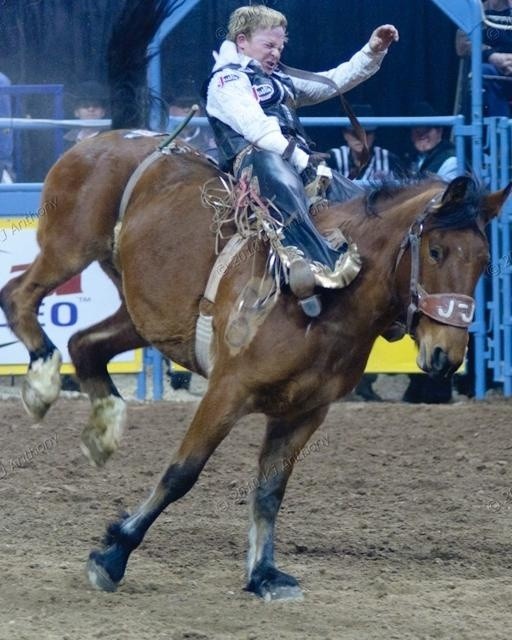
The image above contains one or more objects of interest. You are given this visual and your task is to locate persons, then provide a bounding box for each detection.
[159,74,222,392]
[400,102,472,408]
[453,1,512,179]
[198,0,400,318]
[60,81,122,391]
[322,102,403,402]
[1,69,18,185]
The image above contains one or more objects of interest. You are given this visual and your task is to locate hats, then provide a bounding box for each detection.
[163,77,202,106]
[63,80,108,109]
[339,103,382,132]
[403,102,446,136]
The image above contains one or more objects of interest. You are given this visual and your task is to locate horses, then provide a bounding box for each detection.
[0,0,512,603]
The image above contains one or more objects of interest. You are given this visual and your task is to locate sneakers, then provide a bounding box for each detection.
[288,259,324,319]
[355,380,379,399]
